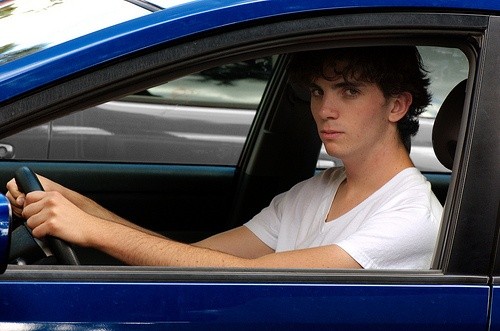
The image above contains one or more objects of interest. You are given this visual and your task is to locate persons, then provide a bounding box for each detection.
[5,39,445,272]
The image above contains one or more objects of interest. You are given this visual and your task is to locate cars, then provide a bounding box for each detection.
[1,1,500,331]
